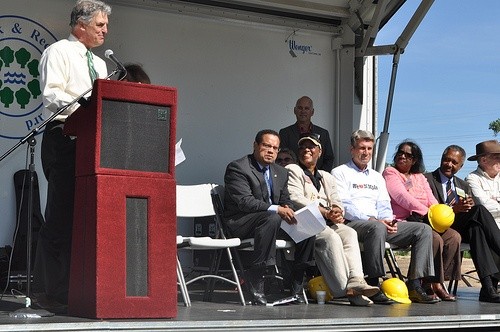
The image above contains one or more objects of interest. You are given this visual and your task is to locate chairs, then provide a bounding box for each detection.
[208,184,309,306]
[453,244,470,296]
[384,242,450,295]
[175,183,246,307]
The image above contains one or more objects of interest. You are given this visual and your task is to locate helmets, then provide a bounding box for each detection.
[428,204,455,233]
[307,276,333,302]
[380,278,412,304]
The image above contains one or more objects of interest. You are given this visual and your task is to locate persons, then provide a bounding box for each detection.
[224,97,380,306]
[382,141,462,302]
[464,140,500,229]
[423,145,500,303]
[330,130,440,304]
[30,0,111,317]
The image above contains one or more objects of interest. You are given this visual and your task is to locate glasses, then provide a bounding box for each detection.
[275,157,294,162]
[260,143,281,152]
[396,149,414,159]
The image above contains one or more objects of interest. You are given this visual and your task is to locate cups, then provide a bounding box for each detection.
[316,290,327,305]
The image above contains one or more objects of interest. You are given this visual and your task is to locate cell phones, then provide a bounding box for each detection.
[391,218,402,226]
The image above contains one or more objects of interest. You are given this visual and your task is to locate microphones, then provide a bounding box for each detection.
[105,49,125,72]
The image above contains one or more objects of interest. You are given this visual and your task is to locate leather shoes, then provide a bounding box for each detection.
[290,279,306,305]
[239,270,267,306]
[428,282,455,301]
[408,287,442,304]
[369,289,394,304]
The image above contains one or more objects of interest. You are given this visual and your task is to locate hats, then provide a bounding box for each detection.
[297,132,322,150]
[467,140,500,161]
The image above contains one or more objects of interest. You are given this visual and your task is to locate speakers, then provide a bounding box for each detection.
[193,216,239,270]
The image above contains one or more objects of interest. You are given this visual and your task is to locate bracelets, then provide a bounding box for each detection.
[324,210,329,220]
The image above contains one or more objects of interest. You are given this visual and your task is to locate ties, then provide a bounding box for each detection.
[446,179,452,203]
[262,167,271,205]
[86,51,99,88]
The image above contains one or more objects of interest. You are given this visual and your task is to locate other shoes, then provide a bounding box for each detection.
[30,292,67,314]
[346,281,380,297]
[479,288,500,303]
[348,295,374,306]
